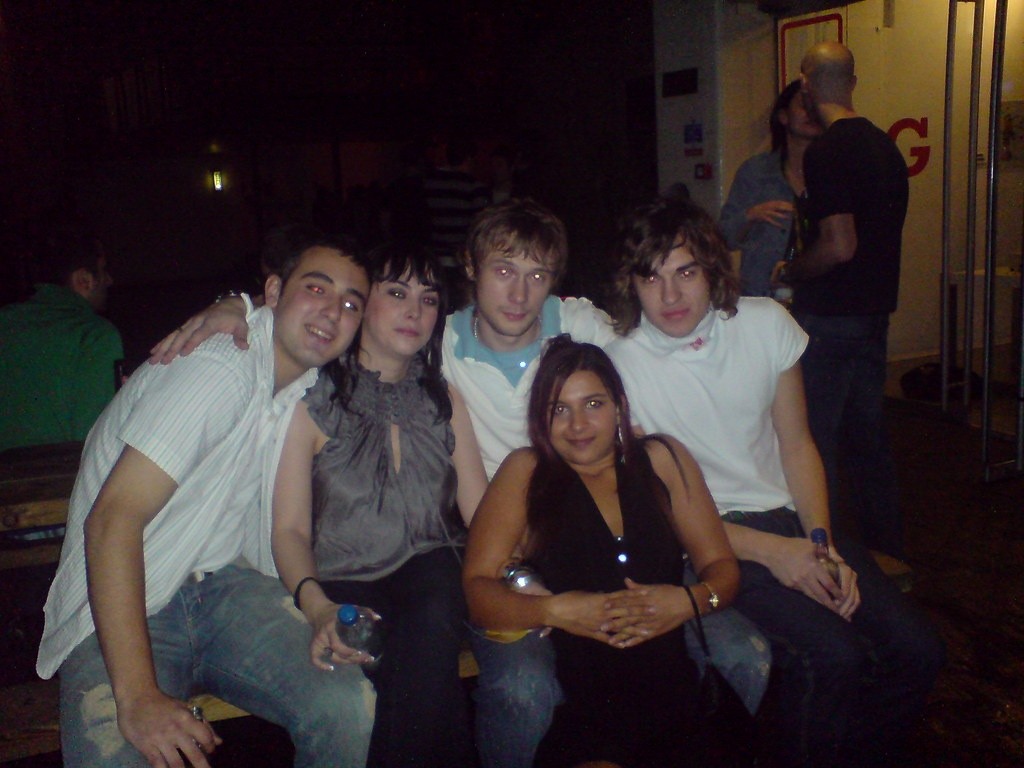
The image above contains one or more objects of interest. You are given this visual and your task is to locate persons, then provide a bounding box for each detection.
[0,40,947,768]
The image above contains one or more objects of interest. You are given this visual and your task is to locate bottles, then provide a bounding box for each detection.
[771,248,795,311]
[337,606,385,673]
[811,528,841,600]
[504,563,555,636]
[181,707,208,759]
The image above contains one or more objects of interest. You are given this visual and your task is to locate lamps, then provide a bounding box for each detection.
[208,144,226,208]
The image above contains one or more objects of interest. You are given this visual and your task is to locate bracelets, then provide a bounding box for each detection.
[697,569,721,612]
[215,289,237,303]
[293,577,322,609]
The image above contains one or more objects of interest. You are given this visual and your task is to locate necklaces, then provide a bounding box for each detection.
[788,164,803,179]
[473,314,541,338]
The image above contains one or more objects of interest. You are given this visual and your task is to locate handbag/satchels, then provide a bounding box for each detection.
[670,585,754,768]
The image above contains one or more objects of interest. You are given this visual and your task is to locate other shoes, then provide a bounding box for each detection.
[882,569,915,592]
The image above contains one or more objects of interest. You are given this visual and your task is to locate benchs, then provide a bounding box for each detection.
[0,440,914,768]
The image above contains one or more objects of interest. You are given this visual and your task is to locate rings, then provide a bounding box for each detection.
[177,327,183,332]
